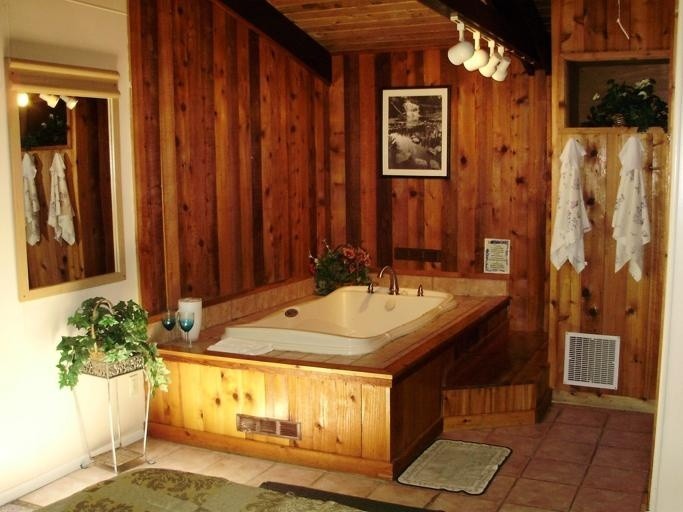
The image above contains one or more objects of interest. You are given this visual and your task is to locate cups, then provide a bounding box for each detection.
[175,298,202,342]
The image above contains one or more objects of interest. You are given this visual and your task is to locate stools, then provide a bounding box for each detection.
[71,370,154,476]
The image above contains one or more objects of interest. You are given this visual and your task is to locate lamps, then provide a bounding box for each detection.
[446,12,511,82]
[39,93,78,109]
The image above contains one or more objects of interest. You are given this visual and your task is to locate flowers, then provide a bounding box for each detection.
[22,114,67,152]
[581,78,668,133]
[308,240,371,296]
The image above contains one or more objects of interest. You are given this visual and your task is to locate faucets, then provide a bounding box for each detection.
[376,264,399,296]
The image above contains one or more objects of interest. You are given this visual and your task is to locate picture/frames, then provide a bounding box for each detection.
[380,85,451,179]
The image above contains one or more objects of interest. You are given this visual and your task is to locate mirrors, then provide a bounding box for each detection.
[3,56,125,303]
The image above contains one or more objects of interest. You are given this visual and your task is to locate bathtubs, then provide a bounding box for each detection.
[223,284,459,357]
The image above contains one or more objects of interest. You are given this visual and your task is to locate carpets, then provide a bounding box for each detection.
[396,438,512,495]
[32,468,367,512]
[259,481,445,512]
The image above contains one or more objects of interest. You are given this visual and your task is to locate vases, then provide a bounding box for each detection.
[611,114,624,127]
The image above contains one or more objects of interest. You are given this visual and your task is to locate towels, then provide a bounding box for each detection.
[207,336,274,356]
[22,153,40,246]
[47,152,75,246]
[612,136,651,282]
[550,138,592,274]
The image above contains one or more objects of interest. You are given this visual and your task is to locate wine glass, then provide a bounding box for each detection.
[160,311,195,349]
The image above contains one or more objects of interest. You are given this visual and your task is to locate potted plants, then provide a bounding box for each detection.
[56,297,172,397]
